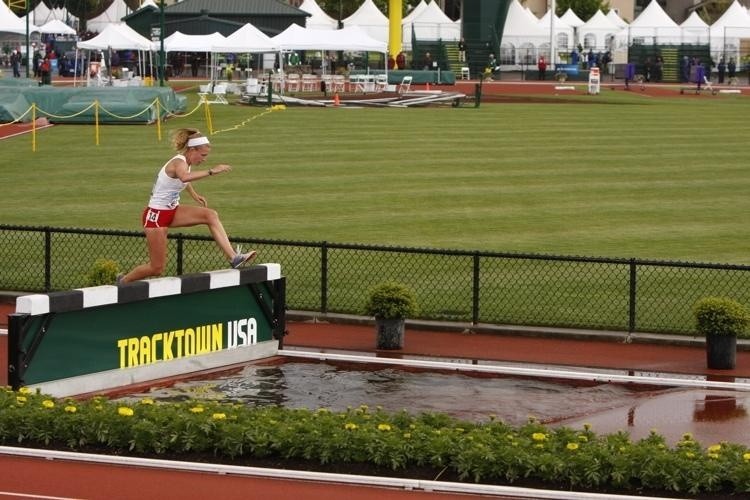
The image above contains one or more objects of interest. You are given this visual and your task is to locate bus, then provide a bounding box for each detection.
[45,35,102,77]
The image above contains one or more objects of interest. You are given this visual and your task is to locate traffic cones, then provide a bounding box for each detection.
[425,81,430,90]
[333,91,340,107]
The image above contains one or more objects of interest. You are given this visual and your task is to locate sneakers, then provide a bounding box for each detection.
[229,250,256,268]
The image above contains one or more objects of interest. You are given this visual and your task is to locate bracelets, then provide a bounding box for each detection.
[208,168,212,175]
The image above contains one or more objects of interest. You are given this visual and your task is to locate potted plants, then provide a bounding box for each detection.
[696,297,750,369]
[365,282,417,347]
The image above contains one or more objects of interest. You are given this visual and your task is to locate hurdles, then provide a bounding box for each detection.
[7,264,286,400]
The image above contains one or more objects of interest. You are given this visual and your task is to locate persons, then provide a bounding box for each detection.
[647,55,664,81]
[115,127,256,285]
[111,51,120,67]
[422,52,432,69]
[388,55,394,69]
[396,51,406,69]
[330,55,337,75]
[458,36,466,62]
[172,52,183,76]
[538,47,613,82]
[681,55,736,84]
[488,54,497,82]
[10,49,69,85]
[191,53,200,76]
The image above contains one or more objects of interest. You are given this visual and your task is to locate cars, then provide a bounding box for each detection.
[57,51,87,76]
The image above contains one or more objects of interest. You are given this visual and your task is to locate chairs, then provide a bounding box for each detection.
[461,67,470,80]
[703,76,713,91]
[197,73,413,105]
